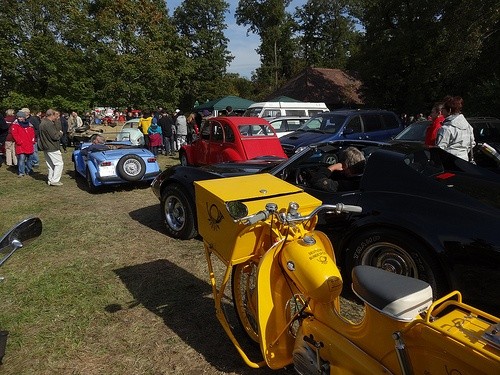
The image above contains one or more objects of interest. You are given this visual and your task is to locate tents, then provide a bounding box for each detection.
[194,94,303,134]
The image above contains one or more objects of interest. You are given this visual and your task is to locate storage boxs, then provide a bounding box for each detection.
[192,171,321,256]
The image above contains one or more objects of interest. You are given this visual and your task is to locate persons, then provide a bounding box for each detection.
[310,147,366,194]
[400,96,476,162]
[0,106,237,186]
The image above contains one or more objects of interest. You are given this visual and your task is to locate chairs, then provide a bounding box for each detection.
[336,160,367,192]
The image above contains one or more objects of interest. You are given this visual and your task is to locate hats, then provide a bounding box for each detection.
[152,118,157,123]
[19,108,30,112]
[203,110,211,117]
[17,112,26,116]
[176,109,180,112]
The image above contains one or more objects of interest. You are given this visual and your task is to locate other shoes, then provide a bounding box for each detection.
[171,154,176,156]
[166,154,171,156]
[48,182,63,185]
[64,150,67,153]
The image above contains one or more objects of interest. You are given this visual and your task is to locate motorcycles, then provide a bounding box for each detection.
[230,201,500,375]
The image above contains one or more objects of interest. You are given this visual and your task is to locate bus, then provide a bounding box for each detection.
[240,101,335,131]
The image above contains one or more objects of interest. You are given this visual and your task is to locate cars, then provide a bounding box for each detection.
[179,116,288,167]
[115,118,146,147]
[278,108,405,159]
[71,137,161,193]
[391,117,500,174]
[149,138,499,320]
[237,115,328,139]
